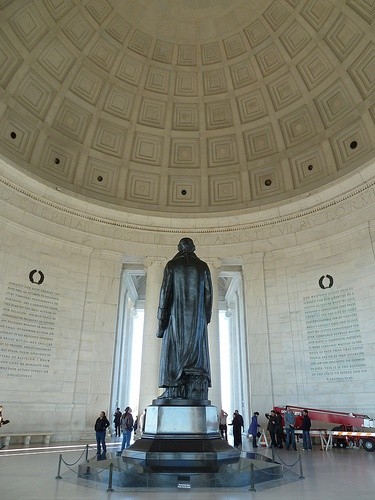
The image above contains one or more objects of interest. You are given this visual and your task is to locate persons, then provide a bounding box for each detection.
[235,410,244,444]
[248,412,261,447]
[156,238,213,399]
[228,413,240,447]
[301,410,312,452]
[272,411,283,449]
[284,406,297,451]
[219,410,228,441]
[134,416,138,431]
[95,411,110,454]
[0,406,6,450]
[121,407,133,451]
[113,408,122,437]
[265,410,276,448]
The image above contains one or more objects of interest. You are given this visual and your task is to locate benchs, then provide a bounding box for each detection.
[0,431,54,446]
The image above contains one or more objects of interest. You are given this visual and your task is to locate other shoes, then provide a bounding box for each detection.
[308,448,311,451]
[302,448,305,451]
[96,452,100,454]
[235,446,237,448]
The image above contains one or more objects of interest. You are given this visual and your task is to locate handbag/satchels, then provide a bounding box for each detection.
[257,432,261,438]
[219,424,226,430]
